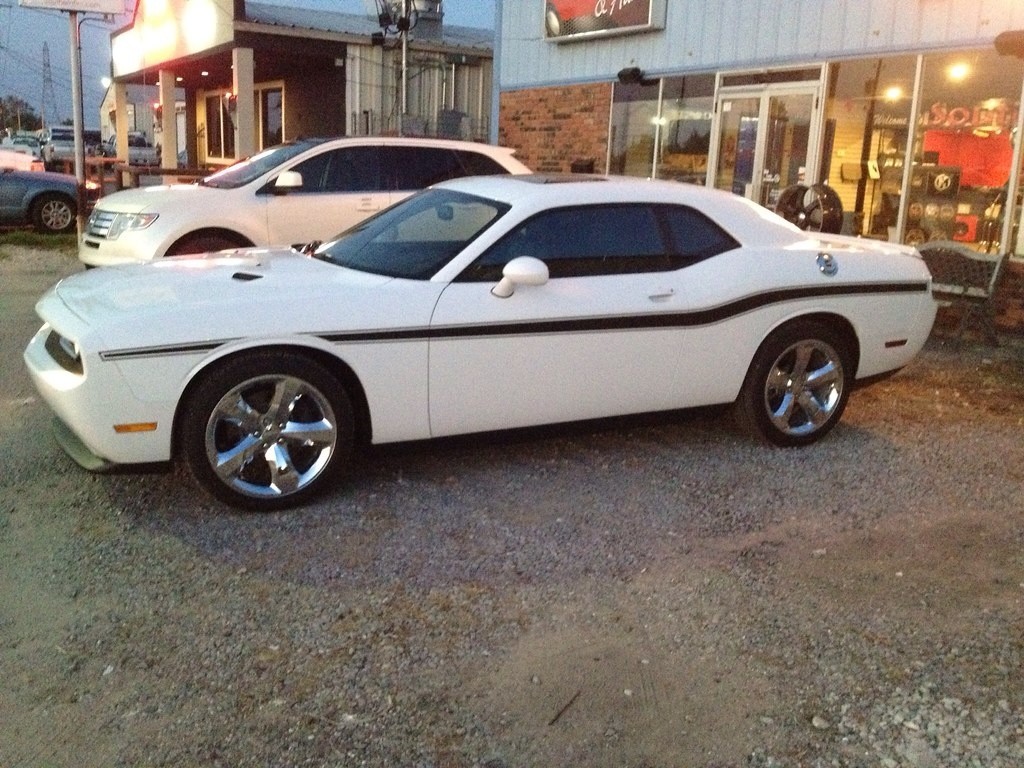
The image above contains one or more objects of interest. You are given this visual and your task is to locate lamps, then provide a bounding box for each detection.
[616,67,644,85]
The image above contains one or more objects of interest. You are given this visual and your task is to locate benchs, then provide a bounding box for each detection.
[907,240,1011,346]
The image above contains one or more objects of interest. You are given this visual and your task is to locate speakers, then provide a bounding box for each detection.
[618,66,641,84]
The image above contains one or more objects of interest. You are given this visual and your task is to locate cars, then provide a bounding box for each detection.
[27,173,939,514]
[0,125,160,173]
[82,138,534,267]
[0,168,82,234]
